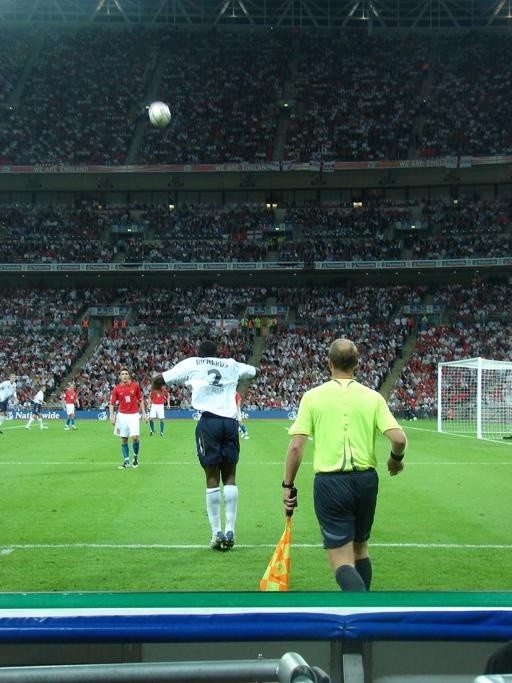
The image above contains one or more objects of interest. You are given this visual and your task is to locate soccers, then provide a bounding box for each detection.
[149,101,171,127]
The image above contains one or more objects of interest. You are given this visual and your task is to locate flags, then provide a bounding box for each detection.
[260,514,290,591]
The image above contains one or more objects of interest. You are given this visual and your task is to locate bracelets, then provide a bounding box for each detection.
[390,451,404,461]
[282,480,293,489]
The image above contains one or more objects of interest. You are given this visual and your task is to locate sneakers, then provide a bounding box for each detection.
[118,457,138,468]
[210,531,234,548]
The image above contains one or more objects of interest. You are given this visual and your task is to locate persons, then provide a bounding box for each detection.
[152,339,261,550]
[486,640,512,675]
[109,369,147,469]
[282,339,407,588]
